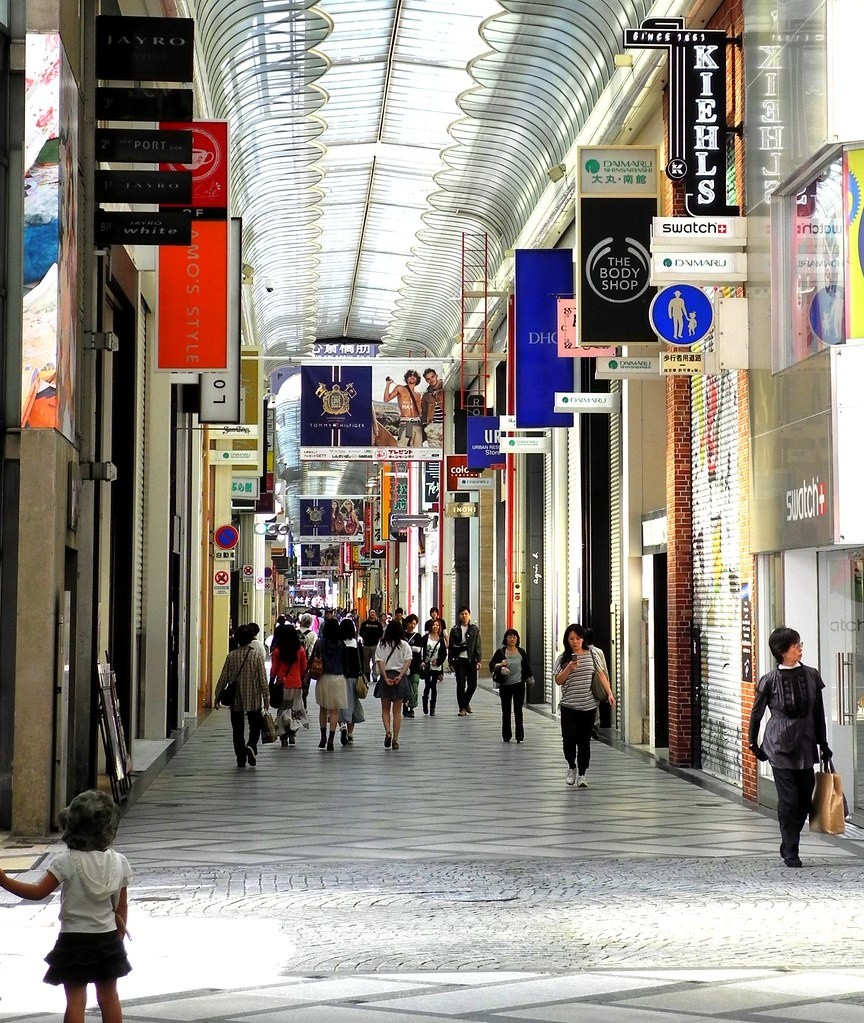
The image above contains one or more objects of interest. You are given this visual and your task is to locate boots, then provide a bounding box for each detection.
[422,696,429,714]
[430,700,437,716]
[318,728,327,748]
[327,731,335,751]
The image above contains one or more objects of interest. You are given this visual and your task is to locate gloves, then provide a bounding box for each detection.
[749,744,768,762]
[820,742,832,761]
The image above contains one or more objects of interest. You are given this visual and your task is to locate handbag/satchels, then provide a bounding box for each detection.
[212,642,618,706]
[260,707,277,744]
[810,755,845,835]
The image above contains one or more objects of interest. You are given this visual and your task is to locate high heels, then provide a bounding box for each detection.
[516,736,523,743]
[503,735,511,741]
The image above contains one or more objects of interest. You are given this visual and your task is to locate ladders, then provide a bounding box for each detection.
[461,231,488,417]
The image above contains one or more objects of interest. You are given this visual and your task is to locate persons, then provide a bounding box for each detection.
[489,629,534,742]
[0,789,133,1023]
[383,368,443,448]
[747,628,833,867]
[338,619,365,744]
[215,625,270,768]
[379,613,392,633]
[268,625,309,745]
[276,607,359,635]
[331,499,361,536]
[295,595,324,608]
[249,623,267,660]
[552,624,616,788]
[316,618,348,751]
[375,620,413,749]
[297,614,318,709]
[325,544,335,566]
[394,607,449,717]
[359,609,384,682]
[448,606,483,716]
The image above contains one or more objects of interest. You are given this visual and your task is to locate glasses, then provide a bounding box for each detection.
[790,641,804,650]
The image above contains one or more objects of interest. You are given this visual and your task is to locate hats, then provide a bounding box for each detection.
[60,788,120,852]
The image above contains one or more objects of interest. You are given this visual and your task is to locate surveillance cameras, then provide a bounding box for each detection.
[264,279,275,292]
[472,281,484,292]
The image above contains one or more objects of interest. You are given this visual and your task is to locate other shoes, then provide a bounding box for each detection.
[289,736,295,747]
[282,740,288,747]
[348,736,354,744]
[392,742,399,749]
[458,708,467,716]
[785,859,803,867]
[341,729,347,745]
[403,701,415,718]
[385,731,391,750]
[467,707,473,714]
[238,760,246,767]
[245,742,257,766]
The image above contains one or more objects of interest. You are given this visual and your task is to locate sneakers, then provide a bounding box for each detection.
[578,775,587,787]
[566,767,576,785]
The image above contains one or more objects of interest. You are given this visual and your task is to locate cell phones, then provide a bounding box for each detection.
[572,654,577,662]
